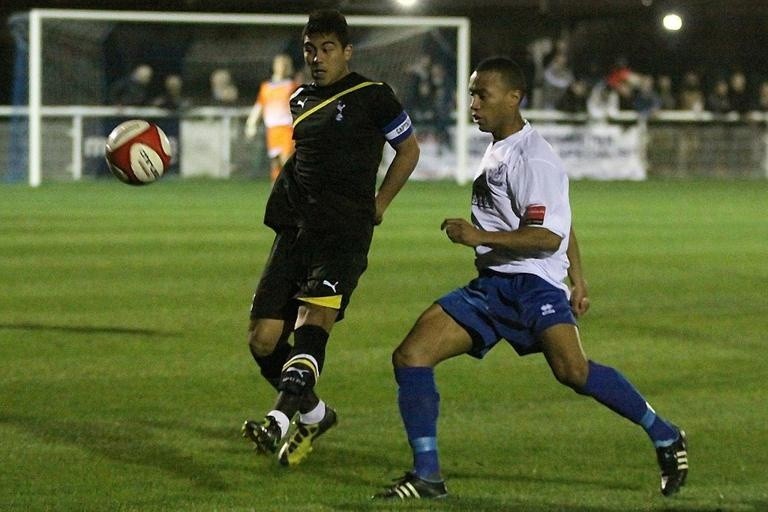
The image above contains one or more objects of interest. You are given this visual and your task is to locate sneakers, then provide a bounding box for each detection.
[655,420,688,497]
[372,471,448,501]
[279,405,339,468]
[242,416,282,455]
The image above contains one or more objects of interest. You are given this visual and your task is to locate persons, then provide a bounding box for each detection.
[94,62,240,179]
[516,34,768,114]
[241,8,419,478]
[391,51,457,157]
[368,54,690,501]
[242,52,303,190]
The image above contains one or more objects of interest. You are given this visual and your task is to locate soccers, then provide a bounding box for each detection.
[106,121,170,184]
